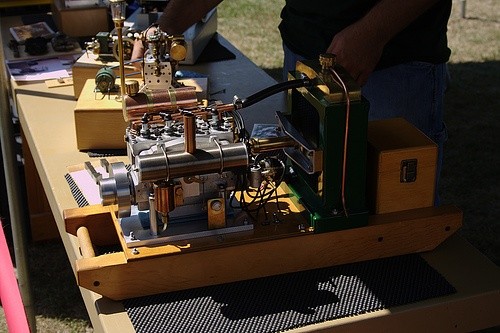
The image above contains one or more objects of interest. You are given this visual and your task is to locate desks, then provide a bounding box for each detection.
[0,0,500,333]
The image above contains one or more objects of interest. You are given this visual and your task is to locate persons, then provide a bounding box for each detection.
[142,0,455,218]
